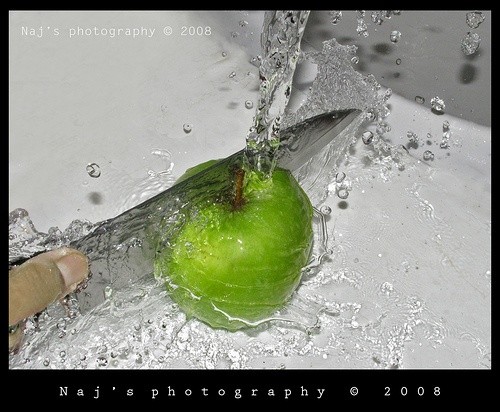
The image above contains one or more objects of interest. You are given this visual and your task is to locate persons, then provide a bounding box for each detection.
[9,247,91,349]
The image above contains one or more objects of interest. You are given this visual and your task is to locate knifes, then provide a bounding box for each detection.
[9,107,366,324]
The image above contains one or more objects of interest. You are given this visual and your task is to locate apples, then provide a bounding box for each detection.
[151,158,316,332]
